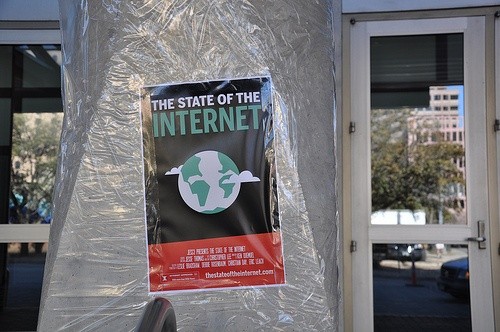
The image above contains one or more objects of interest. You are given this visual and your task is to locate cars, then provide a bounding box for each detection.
[438,256,470,297]
[371,209,426,264]
